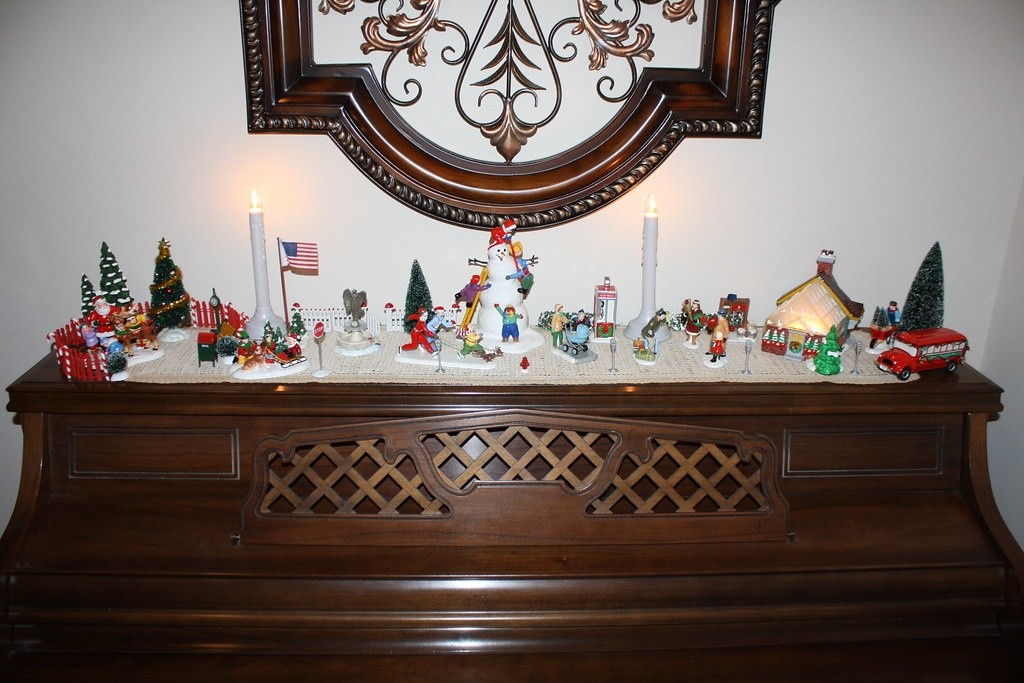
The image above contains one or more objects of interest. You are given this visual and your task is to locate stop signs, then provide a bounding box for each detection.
[313,322,325,338]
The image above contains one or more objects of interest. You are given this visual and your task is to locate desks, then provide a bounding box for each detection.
[0,327,1024,683]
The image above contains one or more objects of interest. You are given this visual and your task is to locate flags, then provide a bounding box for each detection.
[278,238,319,277]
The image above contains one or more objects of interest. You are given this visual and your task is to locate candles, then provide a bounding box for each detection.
[624,192,672,343]
[242,188,288,340]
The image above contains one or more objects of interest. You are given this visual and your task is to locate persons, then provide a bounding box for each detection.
[78,293,159,359]
[234,220,900,364]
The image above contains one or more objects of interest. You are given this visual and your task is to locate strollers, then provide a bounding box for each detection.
[564,320,590,355]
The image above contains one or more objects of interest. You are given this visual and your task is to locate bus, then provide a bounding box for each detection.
[875,327,970,381]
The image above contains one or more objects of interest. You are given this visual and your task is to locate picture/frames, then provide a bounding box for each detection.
[238,0,781,234]
